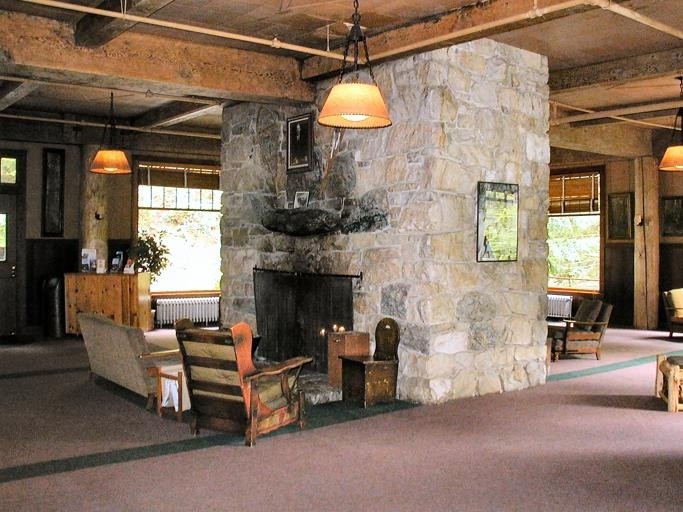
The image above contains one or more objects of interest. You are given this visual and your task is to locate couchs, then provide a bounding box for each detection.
[77,313,183,411]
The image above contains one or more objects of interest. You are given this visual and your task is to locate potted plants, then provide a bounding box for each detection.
[126,229,173,328]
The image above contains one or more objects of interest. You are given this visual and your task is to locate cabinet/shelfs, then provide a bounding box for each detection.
[25,238,82,326]
[327,331,369,390]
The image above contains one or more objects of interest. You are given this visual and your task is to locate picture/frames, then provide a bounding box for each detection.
[287,113,312,174]
[477,181,519,262]
[41,147,65,237]
[661,196,683,237]
[607,193,633,240]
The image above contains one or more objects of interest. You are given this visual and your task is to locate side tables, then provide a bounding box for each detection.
[338,355,399,410]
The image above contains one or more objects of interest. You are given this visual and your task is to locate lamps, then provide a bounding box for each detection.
[88,92,133,174]
[657,76,683,172]
[317,0,392,129]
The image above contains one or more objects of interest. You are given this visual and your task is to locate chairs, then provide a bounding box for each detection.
[176,318,313,448]
[548,299,613,361]
[662,288,683,337]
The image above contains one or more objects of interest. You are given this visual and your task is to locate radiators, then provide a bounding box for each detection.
[547,294,573,318]
[156,297,220,328]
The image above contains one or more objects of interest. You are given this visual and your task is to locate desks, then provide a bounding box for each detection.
[64,272,155,336]
